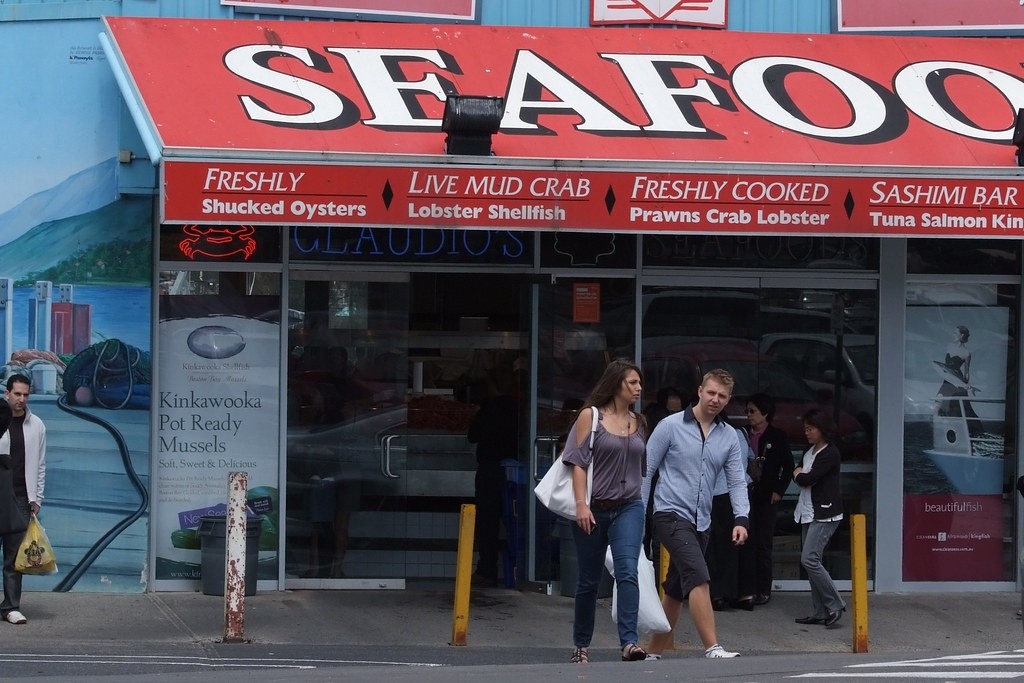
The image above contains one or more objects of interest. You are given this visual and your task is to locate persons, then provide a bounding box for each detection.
[561,359,647,664]
[0,374,47,625]
[641,387,846,627]
[641,370,751,661]
[290,343,549,590]
[935,326,985,438]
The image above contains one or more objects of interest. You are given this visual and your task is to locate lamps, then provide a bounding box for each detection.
[439,93,505,157]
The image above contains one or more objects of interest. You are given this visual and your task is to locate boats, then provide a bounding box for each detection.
[918,395,1005,497]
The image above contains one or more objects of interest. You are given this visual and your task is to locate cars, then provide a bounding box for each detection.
[291,321,879,527]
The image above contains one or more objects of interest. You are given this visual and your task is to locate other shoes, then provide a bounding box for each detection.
[758,593,770,604]
[470,571,497,584]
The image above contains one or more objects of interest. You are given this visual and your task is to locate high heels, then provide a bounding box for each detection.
[825,606,846,626]
[729,597,755,610]
[713,598,726,610]
[795,615,827,623]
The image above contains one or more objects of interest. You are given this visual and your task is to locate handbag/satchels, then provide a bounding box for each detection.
[534,406,599,523]
[604,543,672,634]
[738,426,764,484]
[14,513,58,575]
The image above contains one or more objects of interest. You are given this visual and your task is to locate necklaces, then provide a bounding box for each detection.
[604,407,630,435]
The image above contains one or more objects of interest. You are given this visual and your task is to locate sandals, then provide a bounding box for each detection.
[571,647,589,664]
[622,645,646,661]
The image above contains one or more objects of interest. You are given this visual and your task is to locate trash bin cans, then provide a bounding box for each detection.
[198,515,265,596]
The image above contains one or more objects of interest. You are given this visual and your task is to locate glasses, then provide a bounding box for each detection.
[744,409,760,414]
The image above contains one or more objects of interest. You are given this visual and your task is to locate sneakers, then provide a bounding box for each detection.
[7,611,27,624]
[705,645,741,659]
[646,654,661,660]
